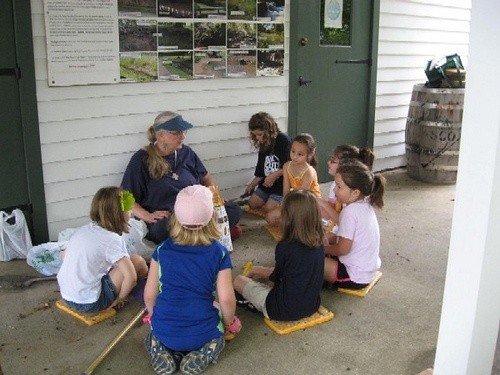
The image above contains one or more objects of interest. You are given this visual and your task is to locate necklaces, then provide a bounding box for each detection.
[165,149,179,180]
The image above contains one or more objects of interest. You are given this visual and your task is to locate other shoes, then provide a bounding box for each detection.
[230,225,243,241]
[179,336,226,375]
[144,333,176,375]
[235,289,261,314]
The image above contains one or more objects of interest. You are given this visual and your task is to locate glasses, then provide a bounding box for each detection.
[329,156,339,164]
[166,129,187,135]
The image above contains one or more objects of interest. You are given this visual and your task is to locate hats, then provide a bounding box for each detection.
[174,185,213,230]
[155,114,193,131]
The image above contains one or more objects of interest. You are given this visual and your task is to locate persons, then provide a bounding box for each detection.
[323,161,386,290]
[316,143,374,245]
[263,133,321,227]
[56,186,149,313]
[233,190,329,322]
[245,111,293,211]
[141,184,242,375]
[120,111,242,244]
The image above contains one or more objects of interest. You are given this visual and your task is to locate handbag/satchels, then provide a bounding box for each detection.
[26,228,80,277]
[121,218,158,263]
[213,204,233,252]
[0,209,32,261]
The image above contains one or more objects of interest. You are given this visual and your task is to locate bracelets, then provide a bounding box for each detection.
[142,313,151,324]
[116,296,129,302]
[275,171,280,179]
[224,316,242,334]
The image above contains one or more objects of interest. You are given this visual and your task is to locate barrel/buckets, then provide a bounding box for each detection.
[405,84,465,185]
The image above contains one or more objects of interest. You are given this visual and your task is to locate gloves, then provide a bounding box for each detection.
[142,314,151,326]
[224,316,242,340]
